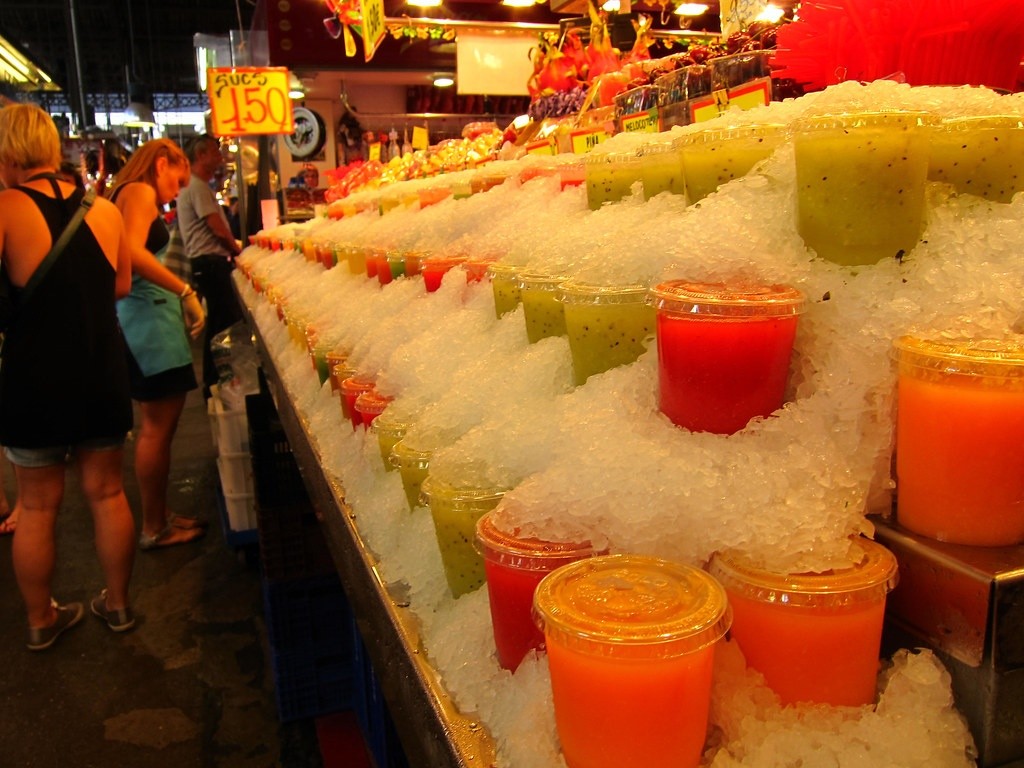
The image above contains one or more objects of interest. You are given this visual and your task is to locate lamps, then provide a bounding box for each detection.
[433,76,454,88]
[122,102,155,127]
[290,73,305,99]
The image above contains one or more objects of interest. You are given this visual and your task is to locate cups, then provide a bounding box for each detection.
[227,110,1024,768]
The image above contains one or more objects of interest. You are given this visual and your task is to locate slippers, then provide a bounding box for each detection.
[0,520,17,534]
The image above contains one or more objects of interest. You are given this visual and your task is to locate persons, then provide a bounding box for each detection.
[0,447,22,533]
[299,164,318,187]
[108,140,206,545]
[0,103,146,647]
[176,135,241,404]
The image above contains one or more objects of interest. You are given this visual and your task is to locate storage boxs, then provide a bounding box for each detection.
[207,367,409,768]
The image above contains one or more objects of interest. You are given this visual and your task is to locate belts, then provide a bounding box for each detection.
[190,255,226,263]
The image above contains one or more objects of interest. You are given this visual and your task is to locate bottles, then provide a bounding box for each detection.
[288,176,298,190]
[388,127,399,160]
[401,127,414,157]
[377,130,388,164]
[298,177,307,189]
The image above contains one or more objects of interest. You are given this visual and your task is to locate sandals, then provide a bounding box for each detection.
[139,527,205,551]
[166,512,205,528]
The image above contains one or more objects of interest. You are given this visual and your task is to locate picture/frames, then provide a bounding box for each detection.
[284,107,320,157]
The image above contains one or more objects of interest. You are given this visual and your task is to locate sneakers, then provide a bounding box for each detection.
[26,597,84,650]
[91,588,136,632]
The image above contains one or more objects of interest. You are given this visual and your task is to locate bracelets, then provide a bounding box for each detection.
[179,284,190,297]
